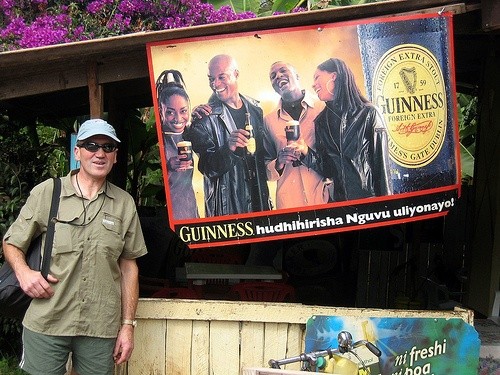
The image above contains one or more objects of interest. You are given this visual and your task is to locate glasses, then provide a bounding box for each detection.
[77,140,117,153]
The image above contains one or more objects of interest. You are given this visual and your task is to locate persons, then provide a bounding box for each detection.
[263,61,334,211]
[293,60,394,202]
[2,119,147,375]
[189,53,274,218]
[157,70,212,221]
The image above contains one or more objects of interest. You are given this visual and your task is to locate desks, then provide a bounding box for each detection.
[183,261,284,288]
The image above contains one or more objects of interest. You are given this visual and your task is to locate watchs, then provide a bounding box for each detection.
[121,319,138,327]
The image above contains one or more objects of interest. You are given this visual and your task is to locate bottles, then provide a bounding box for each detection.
[315,354,358,375]
[243,112,257,157]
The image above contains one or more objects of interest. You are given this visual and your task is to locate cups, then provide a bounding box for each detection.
[285,120,304,168]
[356,17,457,195]
[177,141,193,169]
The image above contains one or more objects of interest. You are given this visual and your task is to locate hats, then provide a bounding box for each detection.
[76,119,122,142]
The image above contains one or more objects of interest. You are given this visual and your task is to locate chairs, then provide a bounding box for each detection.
[231,281,296,303]
[150,287,203,299]
[190,246,244,265]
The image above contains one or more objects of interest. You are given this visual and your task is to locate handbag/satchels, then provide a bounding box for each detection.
[0,229,41,318]
